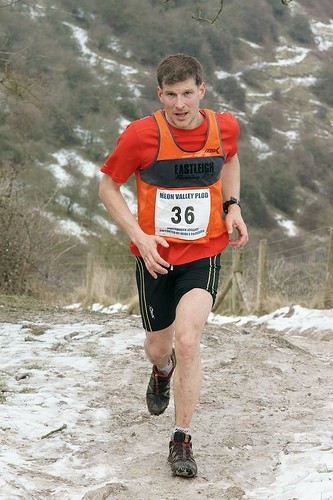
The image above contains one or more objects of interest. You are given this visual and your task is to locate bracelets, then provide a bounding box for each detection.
[223,196,242,214]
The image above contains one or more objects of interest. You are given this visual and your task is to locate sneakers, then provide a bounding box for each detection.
[146,348,177,416]
[168,432,198,478]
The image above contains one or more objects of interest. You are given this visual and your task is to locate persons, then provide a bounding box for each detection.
[98,55,250,477]
